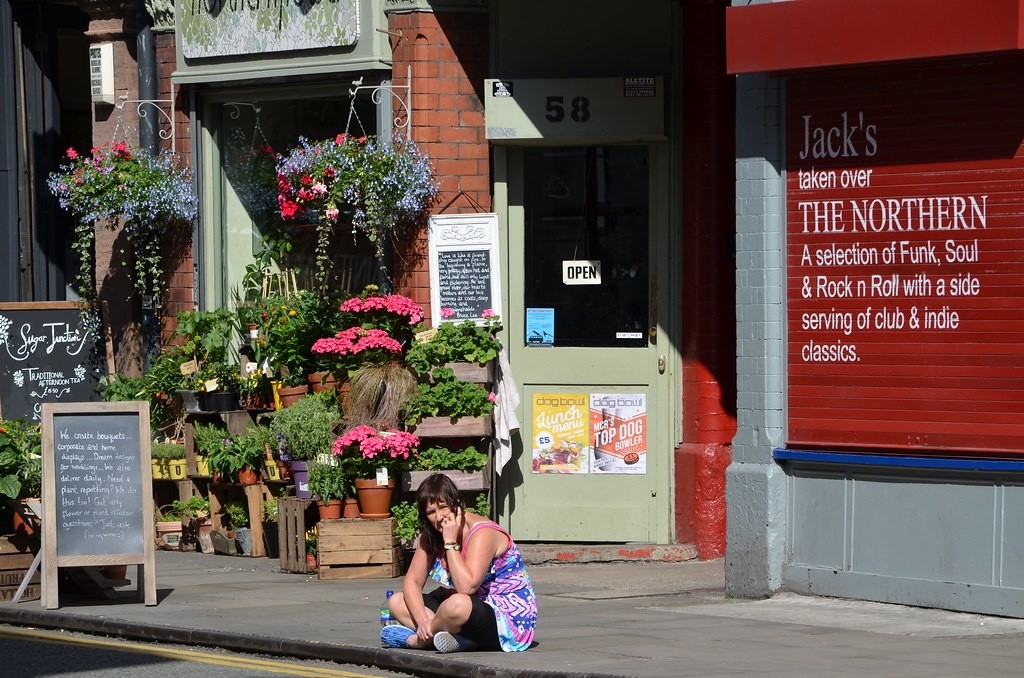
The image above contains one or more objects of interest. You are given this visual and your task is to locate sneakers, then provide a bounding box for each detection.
[433,632,478,653]
[381,625,417,649]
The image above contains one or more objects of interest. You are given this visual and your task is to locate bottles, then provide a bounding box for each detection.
[379,590,400,627]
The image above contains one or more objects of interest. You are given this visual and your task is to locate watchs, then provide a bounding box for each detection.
[444,542,461,550]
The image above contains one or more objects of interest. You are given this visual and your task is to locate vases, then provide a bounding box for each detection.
[425,352,495,385]
[245,394,272,407]
[310,369,346,403]
[101,565,128,581]
[409,407,491,438]
[264,424,280,481]
[196,392,241,412]
[358,477,395,518]
[339,377,369,420]
[306,553,316,568]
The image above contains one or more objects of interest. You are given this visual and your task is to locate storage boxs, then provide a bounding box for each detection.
[175,480,204,551]
[316,521,402,578]
[207,481,280,557]
[279,498,316,573]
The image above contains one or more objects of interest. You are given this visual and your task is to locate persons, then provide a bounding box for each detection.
[381,473,538,652]
[587,209,647,309]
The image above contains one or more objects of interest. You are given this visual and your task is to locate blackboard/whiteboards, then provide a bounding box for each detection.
[437,249,494,320]
[39,401,154,566]
[0,299,119,424]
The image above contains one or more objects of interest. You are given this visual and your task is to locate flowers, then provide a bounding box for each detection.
[223,131,277,223]
[47,142,199,334]
[276,134,436,280]
[199,359,241,392]
[295,527,317,552]
[242,293,498,463]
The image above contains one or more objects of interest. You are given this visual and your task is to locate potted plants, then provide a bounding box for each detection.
[284,417,314,500]
[0,420,41,538]
[236,429,262,484]
[247,303,264,341]
[409,447,493,491]
[344,474,357,517]
[151,444,169,479]
[195,422,209,477]
[187,497,214,554]
[210,427,229,484]
[155,501,185,550]
[169,444,187,480]
[278,312,307,410]
[262,498,281,558]
[223,499,253,556]
[313,468,342,519]
[176,338,197,412]
